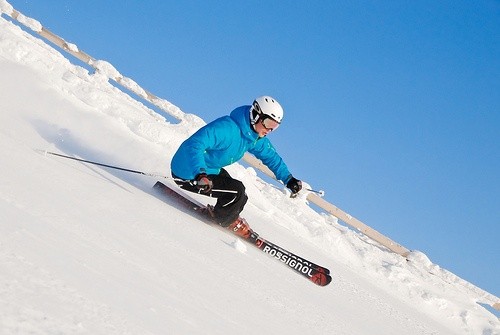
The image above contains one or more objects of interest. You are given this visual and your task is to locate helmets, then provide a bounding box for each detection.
[250,96,283,130]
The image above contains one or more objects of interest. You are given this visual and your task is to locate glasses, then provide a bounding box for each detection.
[253,100,281,132]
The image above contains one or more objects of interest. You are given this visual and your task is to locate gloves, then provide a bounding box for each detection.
[195,173,214,194]
[286,177,302,194]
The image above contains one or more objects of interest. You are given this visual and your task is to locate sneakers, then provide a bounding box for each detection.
[213,208,250,238]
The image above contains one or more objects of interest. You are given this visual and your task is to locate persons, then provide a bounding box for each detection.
[170,96,303,241]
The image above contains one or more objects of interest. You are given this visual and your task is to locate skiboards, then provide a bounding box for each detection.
[152,180,332,287]
[173,187,330,275]
